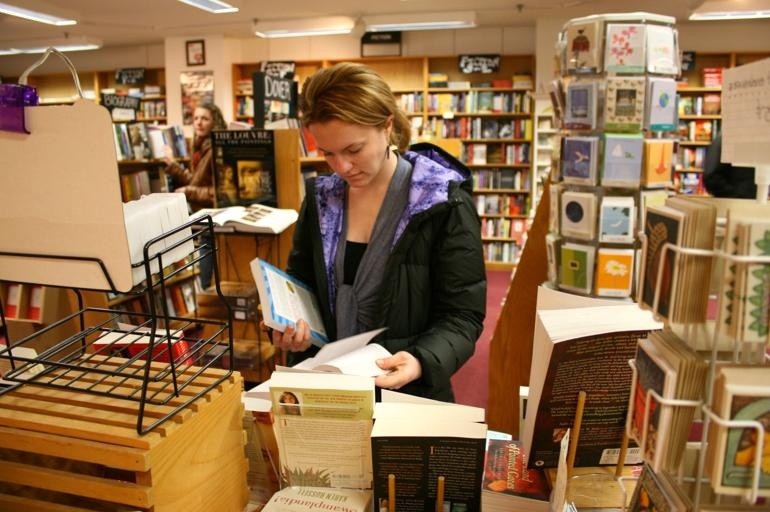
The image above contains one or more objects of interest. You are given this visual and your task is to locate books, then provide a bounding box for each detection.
[0,52,769,512]
